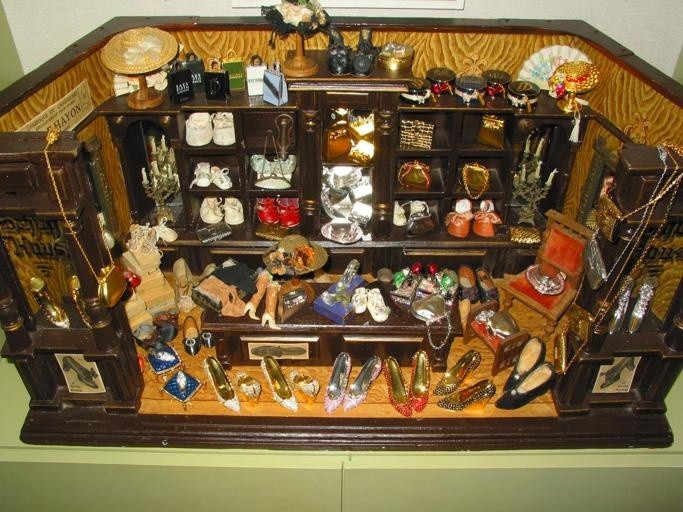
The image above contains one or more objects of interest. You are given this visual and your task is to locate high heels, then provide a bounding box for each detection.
[432,350,482,396]
[289,370,321,405]
[437,378,497,412]
[234,369,262,408]
[600,356,636,390]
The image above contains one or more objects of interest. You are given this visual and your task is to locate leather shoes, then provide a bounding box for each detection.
[259,356,299,412]
[324,352,352,413]
[382,355,412,417]
[495,337,555,410]
[181,310,217,357]
[458,264,500,311]
[344,355,384,410]
[61,355,99,391]
[204,356,242,413]
[409,350,432,411]
[446,197,500,239]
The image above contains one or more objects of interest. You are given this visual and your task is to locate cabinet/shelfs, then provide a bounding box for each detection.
[0,80,682,451]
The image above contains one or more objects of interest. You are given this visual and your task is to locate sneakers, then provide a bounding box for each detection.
[186,109,244,225]
[349,286,391,325]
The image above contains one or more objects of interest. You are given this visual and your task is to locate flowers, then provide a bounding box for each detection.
[274,0,326,30]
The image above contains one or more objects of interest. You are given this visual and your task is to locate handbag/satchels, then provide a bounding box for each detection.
[554,194,624,378]
[412,294,454,353]
[162,43,290,108]
[400,111,508,201]
